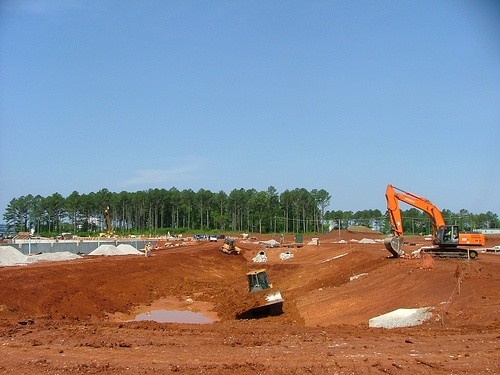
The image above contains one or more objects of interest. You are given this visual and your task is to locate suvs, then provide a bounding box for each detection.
[210,235,217,241]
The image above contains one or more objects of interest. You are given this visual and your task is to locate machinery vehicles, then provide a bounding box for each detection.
[100,206,116,238]
[383,183,485,261]
[237,268,284,317]
[219,237,241,255]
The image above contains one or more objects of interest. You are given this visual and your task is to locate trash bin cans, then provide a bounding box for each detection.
[295,234,304,243]
